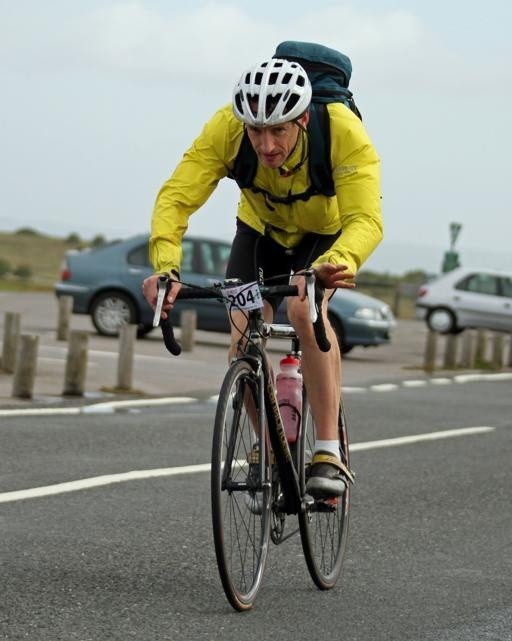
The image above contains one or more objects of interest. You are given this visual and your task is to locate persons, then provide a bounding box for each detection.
[138,53,386,515]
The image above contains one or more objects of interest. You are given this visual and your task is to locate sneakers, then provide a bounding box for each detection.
[244,444,276,515]
[305,450,355,498]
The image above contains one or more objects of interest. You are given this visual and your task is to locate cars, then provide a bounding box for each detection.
[54,232,399,360]
[413,263,512,337]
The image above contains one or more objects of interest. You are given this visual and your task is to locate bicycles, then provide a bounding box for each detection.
[157,261,352,608]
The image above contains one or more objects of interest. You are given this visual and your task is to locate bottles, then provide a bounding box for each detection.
[274,352,305,444]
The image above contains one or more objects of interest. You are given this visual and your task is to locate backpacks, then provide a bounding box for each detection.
[227,41,363,211]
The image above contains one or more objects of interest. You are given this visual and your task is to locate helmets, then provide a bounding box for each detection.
[232,58,313,129]
